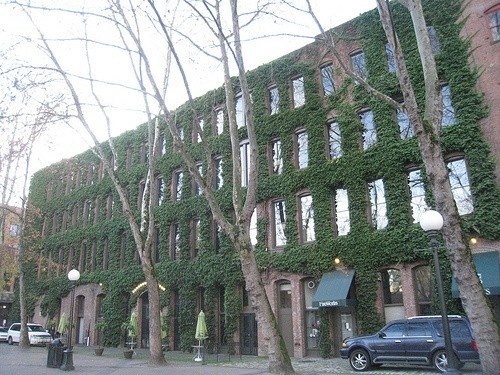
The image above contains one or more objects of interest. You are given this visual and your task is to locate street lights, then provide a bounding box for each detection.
[61,269,80,370]
[420,210,460,375]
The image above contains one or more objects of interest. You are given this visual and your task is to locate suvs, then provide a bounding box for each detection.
[340,315,481,375]
[0,323,54,347]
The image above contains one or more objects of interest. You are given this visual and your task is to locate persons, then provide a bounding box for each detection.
[47,317,56,339]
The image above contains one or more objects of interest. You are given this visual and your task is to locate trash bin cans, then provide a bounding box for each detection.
[45,341,64,368]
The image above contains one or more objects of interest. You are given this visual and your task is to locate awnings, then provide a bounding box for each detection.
[312,270,357,307]
[450,251,500,299]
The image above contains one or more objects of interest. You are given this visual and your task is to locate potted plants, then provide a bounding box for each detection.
[93,322,108,356]
[121,320,135,359]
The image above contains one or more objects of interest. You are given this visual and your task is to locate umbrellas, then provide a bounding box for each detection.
[56,312,68,337]
[160,310,167,342]
[128,313,138,343]
[195,310,209,346]
